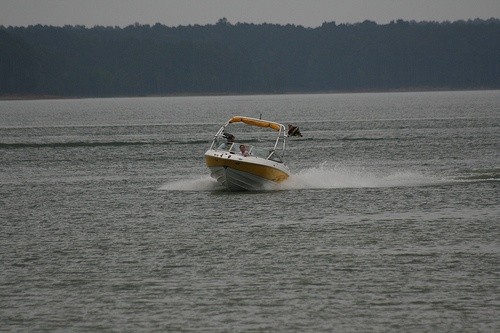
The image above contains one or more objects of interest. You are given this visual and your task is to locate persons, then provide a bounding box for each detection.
[238,144,250,156]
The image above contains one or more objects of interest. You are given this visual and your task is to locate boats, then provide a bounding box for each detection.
[206,115,291,189]
